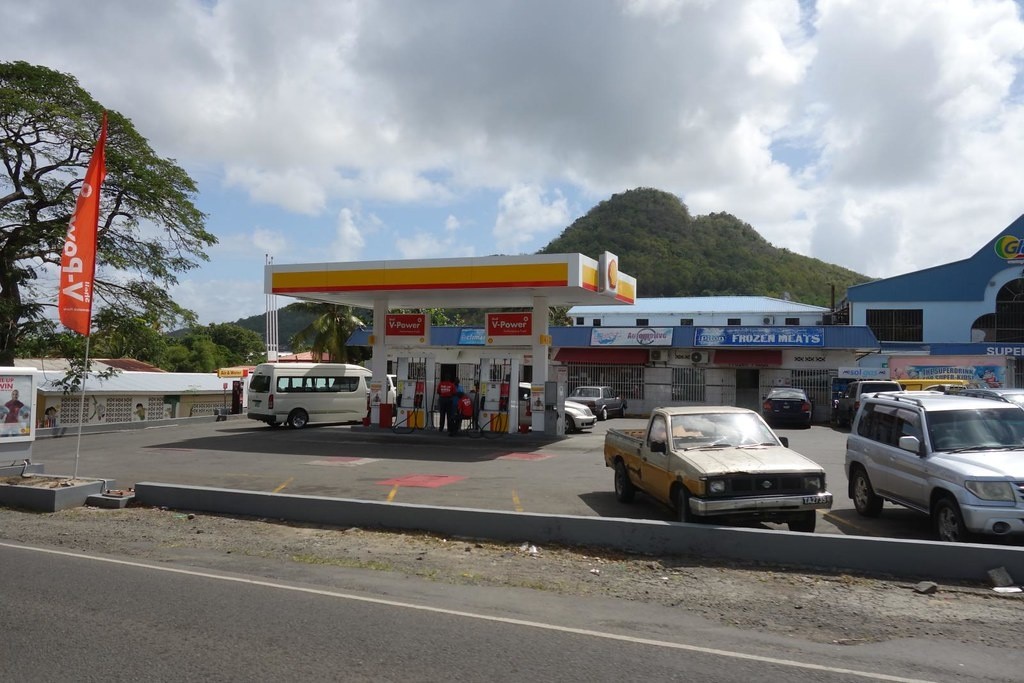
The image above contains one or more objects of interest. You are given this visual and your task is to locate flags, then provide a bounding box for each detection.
[58,111,109,337]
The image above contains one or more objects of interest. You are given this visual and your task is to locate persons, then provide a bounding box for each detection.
[1,389,31,423]
[535,397,543,406]
[449,391,474,437]
[437,374,457,431]
[373,393,381,402]
[451,377,464,431]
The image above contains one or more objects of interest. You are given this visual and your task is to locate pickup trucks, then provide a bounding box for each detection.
[604,406,833,533]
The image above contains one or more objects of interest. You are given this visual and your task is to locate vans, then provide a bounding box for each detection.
[247,362,398,429]
[834,377,1024,543]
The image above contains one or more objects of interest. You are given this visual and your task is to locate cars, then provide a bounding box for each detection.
[566,385,628,421]
[762,388,814,427]
[517,382,597,434]
